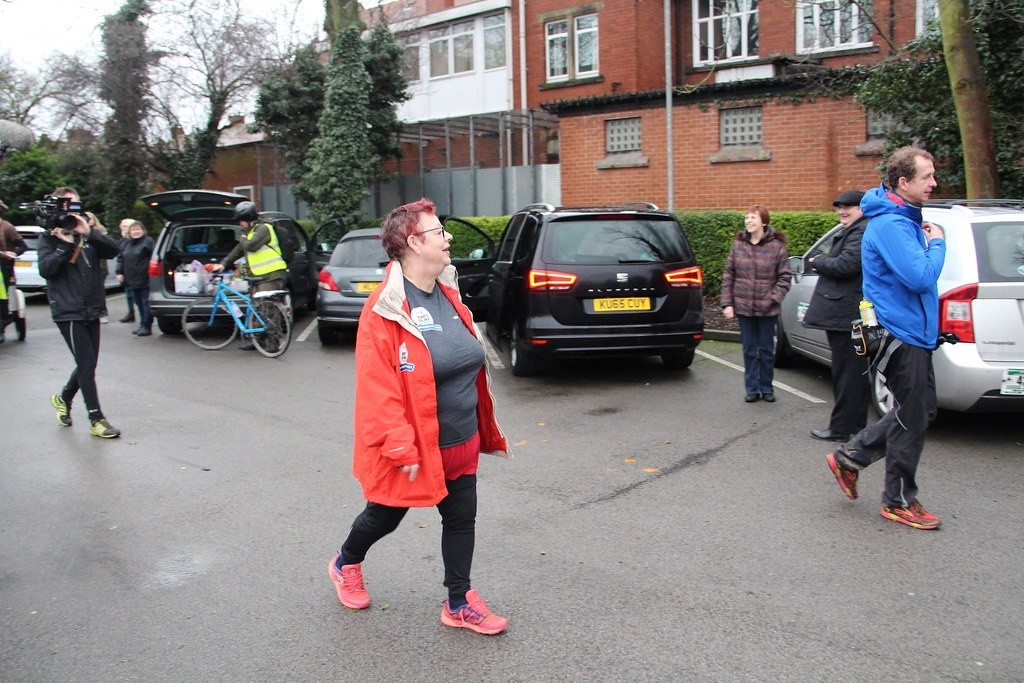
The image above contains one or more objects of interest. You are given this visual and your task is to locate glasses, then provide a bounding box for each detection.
[406,226,445,246]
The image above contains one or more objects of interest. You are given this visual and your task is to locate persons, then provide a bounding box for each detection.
[37,187,121,440]
[802,190,872,442]
[720,205,792,403]
[0,200,29,343]
[825,148,947,529]
[327,197,510,636]
[121,221,156,337]
[114,218,139,323]
[209,201,288,352]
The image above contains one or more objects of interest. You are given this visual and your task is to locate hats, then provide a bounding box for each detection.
[833,190,865,206]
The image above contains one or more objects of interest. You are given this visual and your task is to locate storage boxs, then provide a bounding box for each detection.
[174,271,249,295]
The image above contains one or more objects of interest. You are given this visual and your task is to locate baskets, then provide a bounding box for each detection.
[175,272,205,294]
[205,274,231,297]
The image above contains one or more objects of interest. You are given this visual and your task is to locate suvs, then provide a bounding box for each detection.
[139,188,336,334]
[434,200,705,377]
[767,199,1024,430]
[10,225,124,300]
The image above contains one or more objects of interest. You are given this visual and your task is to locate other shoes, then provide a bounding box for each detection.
[100,317,109,324]
[120,315,134,322]
[266,341,278,352]
[133,328,141,334]
[241,342,262,350]
[138,328,152,336]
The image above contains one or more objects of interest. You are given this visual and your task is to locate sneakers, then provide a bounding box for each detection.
[90,417,121,438]
[745,394,760,403]
[763,393,776,402]
[881,499,941,529]
[327,549,370,609]
[51,393,72,427]
[826,453,859,500]
[441,590,508,635]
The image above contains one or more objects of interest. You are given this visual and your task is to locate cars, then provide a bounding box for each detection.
[315,227,391,344]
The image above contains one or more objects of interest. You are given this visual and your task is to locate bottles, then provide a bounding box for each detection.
[859,300,878,327]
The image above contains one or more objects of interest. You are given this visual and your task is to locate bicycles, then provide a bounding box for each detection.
[181,262,293,357]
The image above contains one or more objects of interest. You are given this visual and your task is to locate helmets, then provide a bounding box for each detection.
[232,201,258,222]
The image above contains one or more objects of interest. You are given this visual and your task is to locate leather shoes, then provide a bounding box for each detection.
[852,428,860,435]
[811,428,850,442]
